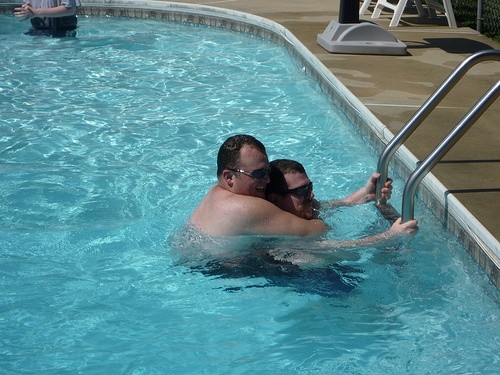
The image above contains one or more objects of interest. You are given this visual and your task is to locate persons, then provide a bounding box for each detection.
[264,160,420,250]
[13,0,81,38]
[189,135,328,238]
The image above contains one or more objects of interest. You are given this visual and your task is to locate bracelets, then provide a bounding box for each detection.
[312,207,320,212]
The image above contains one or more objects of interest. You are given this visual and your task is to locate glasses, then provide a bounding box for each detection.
[226,166,272,179]
[279,182,313,197]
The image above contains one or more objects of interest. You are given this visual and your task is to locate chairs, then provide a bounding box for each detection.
[359,0,458,28]
